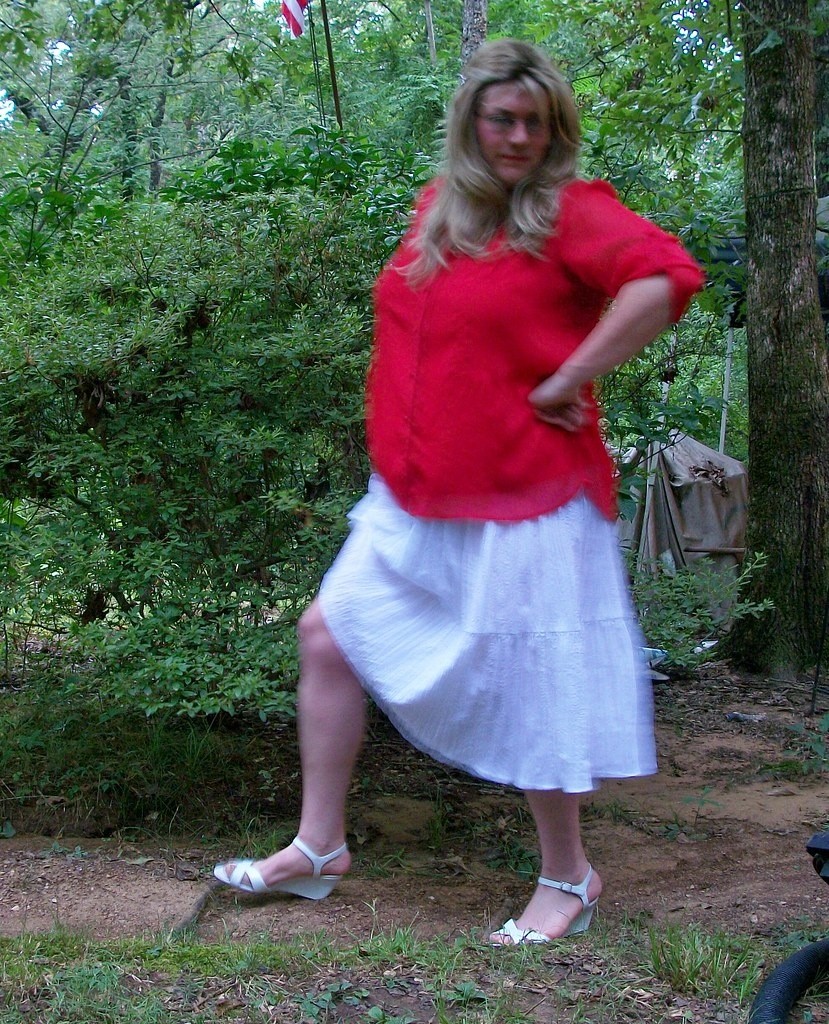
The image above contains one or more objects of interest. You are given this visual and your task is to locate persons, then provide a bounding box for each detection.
[213,40,705,946]
[596,418,625,458]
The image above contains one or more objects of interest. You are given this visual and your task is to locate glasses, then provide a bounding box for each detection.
[474,109,550,135]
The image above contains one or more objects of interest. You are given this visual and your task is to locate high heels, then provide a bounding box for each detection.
[488,862,598,946]
[212,837,348,900]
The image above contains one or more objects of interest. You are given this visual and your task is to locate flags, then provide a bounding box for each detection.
[281,0,308,40]
